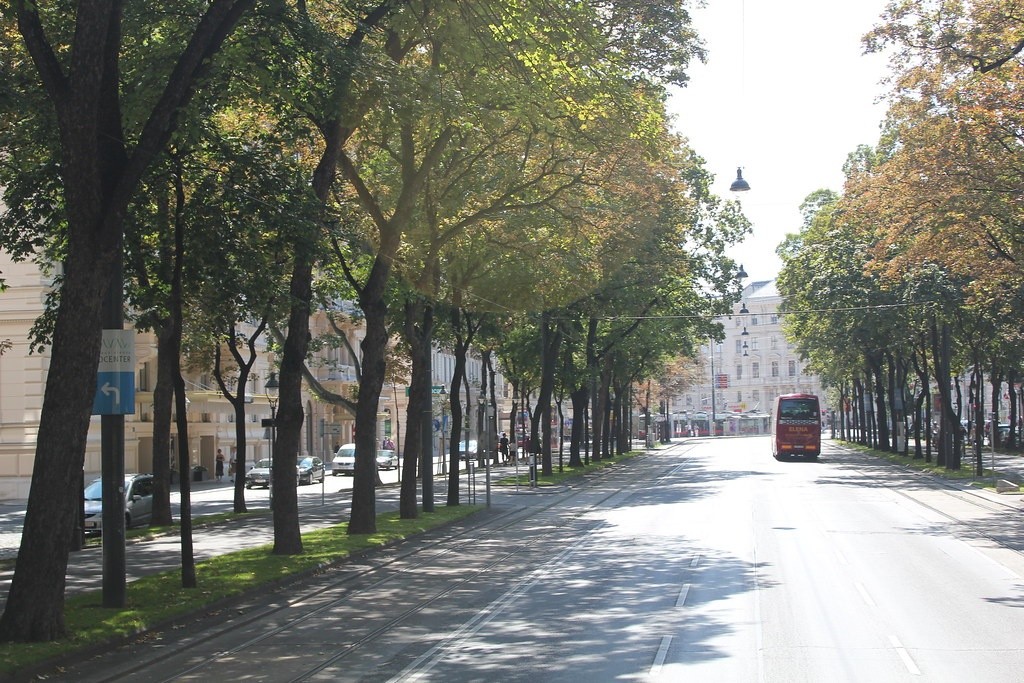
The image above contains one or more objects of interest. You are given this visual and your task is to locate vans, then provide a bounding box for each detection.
[331,443,362,477]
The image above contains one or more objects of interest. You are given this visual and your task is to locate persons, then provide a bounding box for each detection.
[375,438,381,449]
[500,433,509,463]
[535,436,542,464]
[216,449,225,482]
[334,441,340,453]
[383,436,388,450]
[899,411,990,462]
[230,447,237,483]
[524,437,530,465]
[670,421,710,438]
[386,437,395,450]
[507,440,517,466]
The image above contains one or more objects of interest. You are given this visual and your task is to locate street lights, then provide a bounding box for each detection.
[438,384,450,474]
[263,372,280,513]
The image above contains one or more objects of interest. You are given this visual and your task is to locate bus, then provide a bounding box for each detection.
[769,393,822,460]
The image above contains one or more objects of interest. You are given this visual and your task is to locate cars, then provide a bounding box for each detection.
[81,473,158,537]
[458,419,531,464]
[245,455,277,490]
[955,419,1023,450]
[296,452,327,485]
[375,448,399,470]
